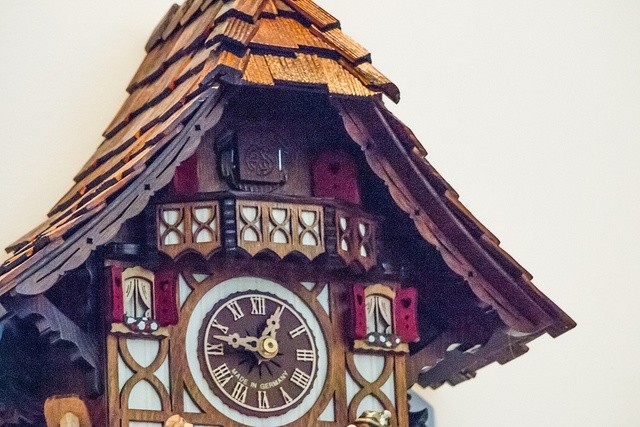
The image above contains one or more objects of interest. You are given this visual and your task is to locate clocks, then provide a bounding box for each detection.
[197,289,319,416]
